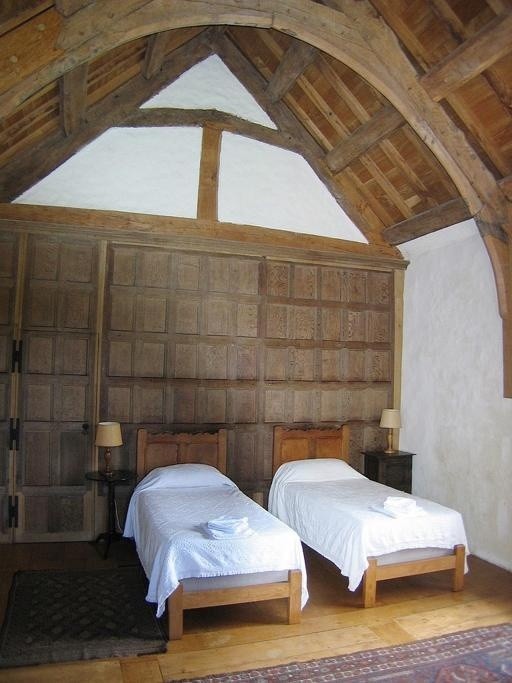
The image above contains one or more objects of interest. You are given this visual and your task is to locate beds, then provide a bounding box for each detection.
[271,423,466,610]
[131,426,304,642]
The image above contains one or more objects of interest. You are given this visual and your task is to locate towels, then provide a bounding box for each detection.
[200,513,257,540]
[373,495,428,521]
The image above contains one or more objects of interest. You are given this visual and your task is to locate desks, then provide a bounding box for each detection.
[85,469,134,559]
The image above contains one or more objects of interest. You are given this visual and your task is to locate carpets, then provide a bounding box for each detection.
[0,561,168,669]
[166,618,511,683]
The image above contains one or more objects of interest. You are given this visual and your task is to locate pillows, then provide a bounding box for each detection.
[285,456,358,480]
[157,461,222,489]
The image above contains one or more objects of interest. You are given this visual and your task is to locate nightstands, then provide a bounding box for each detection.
[358,447,419,495]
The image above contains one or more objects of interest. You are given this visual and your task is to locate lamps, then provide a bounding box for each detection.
[376,405,404,455]
[92,419,128,478]
